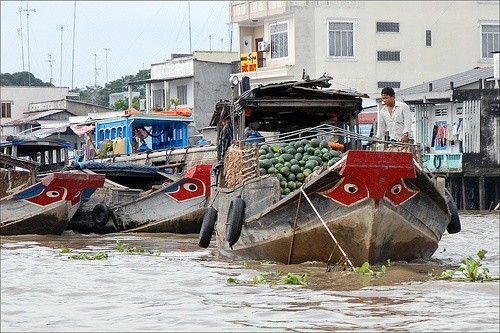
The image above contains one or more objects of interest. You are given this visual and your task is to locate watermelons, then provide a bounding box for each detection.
[252,138,344,196]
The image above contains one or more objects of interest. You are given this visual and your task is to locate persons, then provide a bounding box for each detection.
[368,85,416,145]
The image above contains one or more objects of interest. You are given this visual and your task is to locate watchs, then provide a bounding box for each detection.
[404,134,408,137]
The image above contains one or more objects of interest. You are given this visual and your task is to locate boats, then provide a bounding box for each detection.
[199,69,462,268]
[70,111,221,233]
[0,137,105,237]
